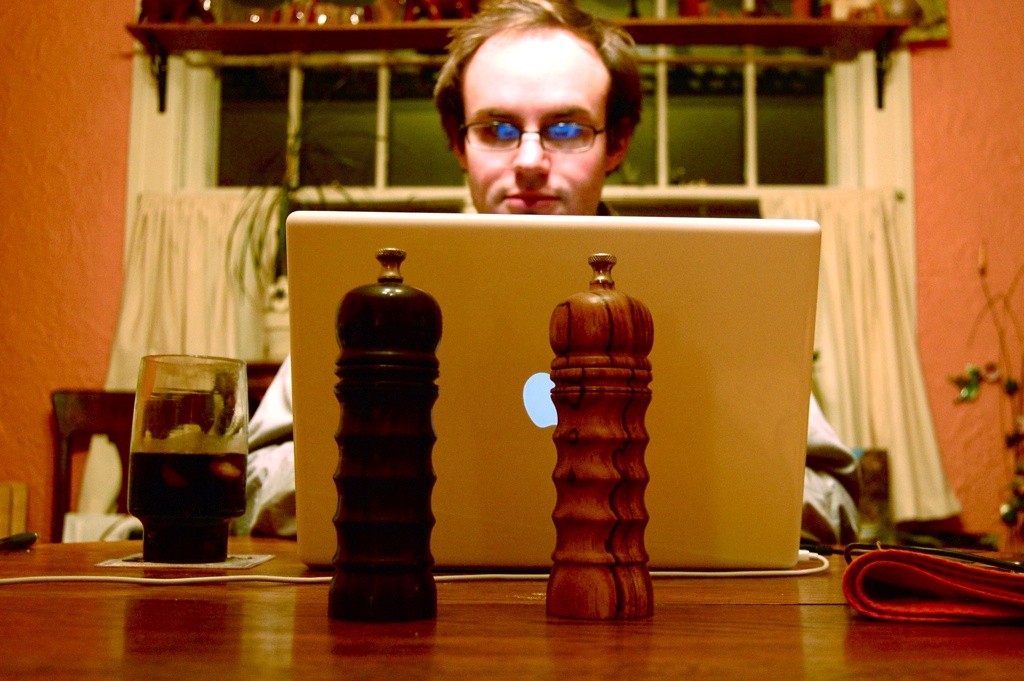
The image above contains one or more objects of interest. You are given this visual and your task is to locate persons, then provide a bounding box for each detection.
[227,0,862,547]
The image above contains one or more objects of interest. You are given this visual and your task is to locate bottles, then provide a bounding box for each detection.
[546,251,656,619]
[329,247,439,622]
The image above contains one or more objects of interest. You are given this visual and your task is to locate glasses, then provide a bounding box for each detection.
[463,116,608,154]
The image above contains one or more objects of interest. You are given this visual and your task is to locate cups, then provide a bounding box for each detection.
[126,353,247,562]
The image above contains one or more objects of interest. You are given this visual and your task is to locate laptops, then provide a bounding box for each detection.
[285,211,823,570]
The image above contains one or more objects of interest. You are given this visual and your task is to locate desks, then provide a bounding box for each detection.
[0,536,1024,681]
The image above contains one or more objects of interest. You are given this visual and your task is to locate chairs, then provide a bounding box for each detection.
[45,388,215,545]
